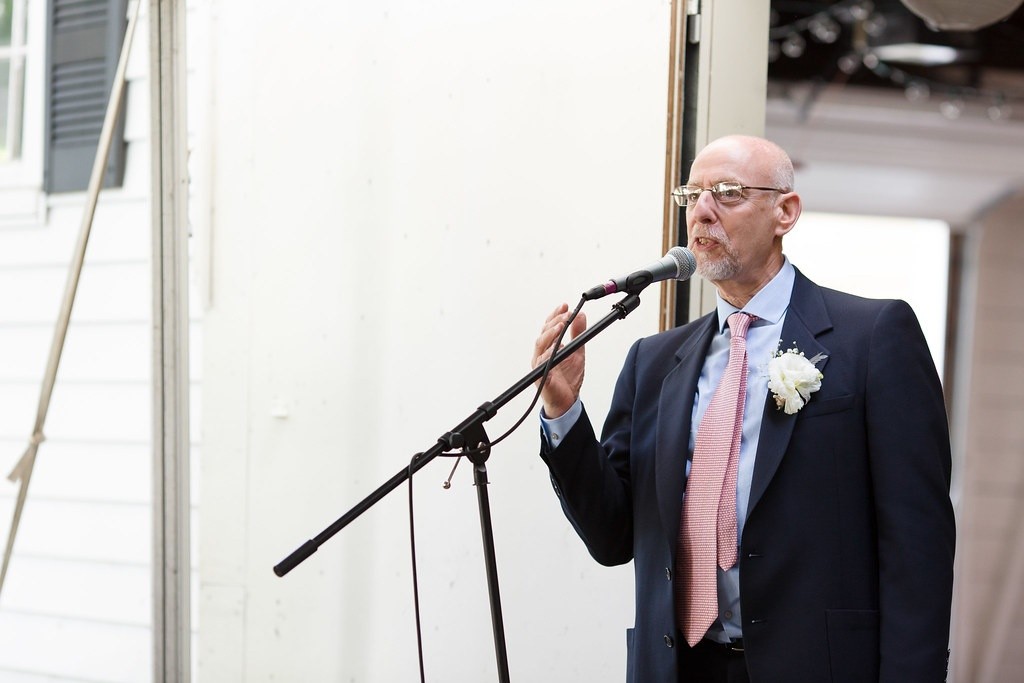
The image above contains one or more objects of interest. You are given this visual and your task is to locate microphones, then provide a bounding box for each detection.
[582,246,697,301]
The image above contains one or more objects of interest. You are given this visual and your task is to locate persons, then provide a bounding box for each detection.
[533,135,956,683]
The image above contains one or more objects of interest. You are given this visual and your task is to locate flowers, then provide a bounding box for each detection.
[757,338,828,415]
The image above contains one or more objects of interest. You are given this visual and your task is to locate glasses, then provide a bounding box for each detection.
[671,181,785,203]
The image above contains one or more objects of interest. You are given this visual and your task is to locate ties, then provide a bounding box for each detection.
[683,314,758,649]
